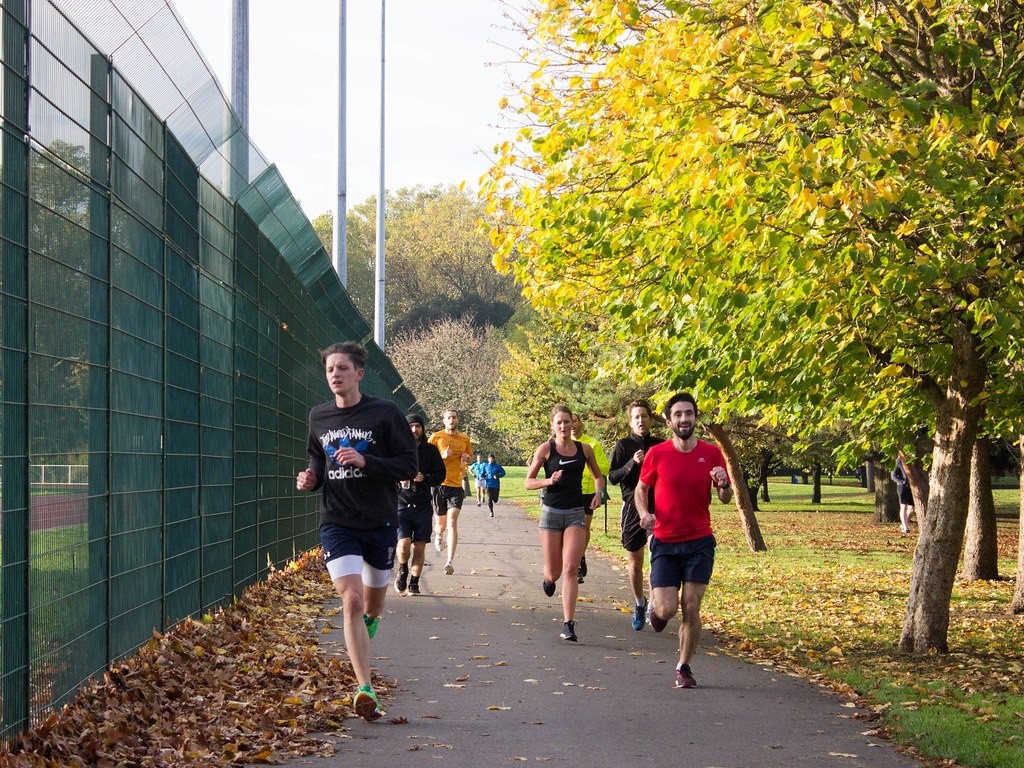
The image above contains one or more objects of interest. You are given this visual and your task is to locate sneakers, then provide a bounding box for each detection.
[675,664,697,689]
[353,687,382,721]
[632,595,647,630]
[394,566,409,593]
[435,533,444,553]
[560,621,578,641]
[408,582,420,597]
[576,567,584,584]
[445,564,454,576]
[580,555,587,576]
[543,578,556,597]
[363,612,378,640]
[648,600,668,633]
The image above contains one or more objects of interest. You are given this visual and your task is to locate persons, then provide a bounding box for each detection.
[608,400,668,632]
[469,454,506,517]
[525,406,605,642]
[427,409,473,575]
[296,341,419,722]
[891,454,915,534]
[568,414,612,583]
[394,414,446,597]
[635,392,733,689]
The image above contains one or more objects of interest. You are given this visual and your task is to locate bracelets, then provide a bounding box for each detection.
[467,455,470,462]
[721,481,731,489]
[596,490,604,496]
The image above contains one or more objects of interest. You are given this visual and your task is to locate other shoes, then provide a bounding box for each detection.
[477,503,481,507]
[490,512,494,518]
[482,498,485,503]
